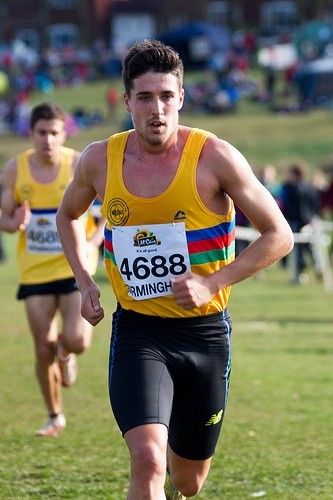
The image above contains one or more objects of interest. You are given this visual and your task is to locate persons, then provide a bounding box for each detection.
[257,159,333,281]
[0,0,333,135]
[0,101,106,436]
[56,39,294,500]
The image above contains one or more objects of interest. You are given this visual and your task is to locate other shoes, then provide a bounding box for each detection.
[163,472,187,500]
[54,335,78,387]
[34,414,66,438]
